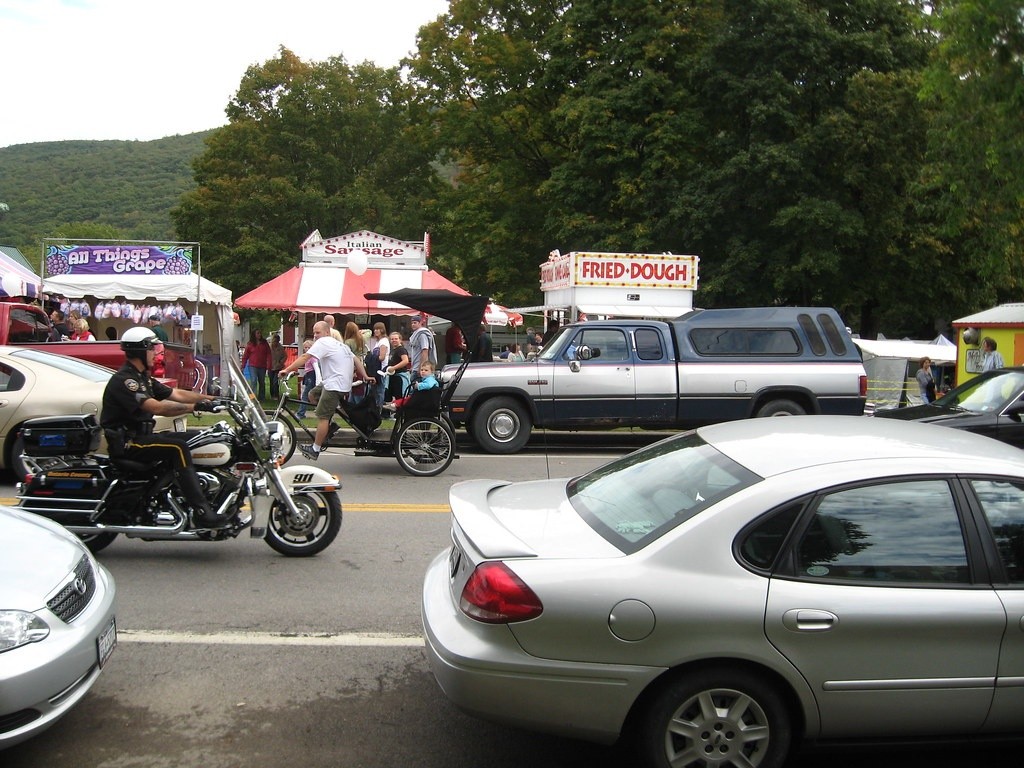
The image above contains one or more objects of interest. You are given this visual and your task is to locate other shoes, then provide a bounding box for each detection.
[325,422,340,438]
[382,404,396,412]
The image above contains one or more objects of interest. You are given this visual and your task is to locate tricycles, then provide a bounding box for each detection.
[247,288,490,476]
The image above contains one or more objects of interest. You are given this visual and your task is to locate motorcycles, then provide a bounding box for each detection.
[15,358,343,556]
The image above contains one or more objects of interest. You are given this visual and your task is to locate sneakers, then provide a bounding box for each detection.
[298,444,319,461]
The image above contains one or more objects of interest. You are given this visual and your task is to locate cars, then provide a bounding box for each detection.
[422,413,1024,768]
[0,345,188,486]
[0,504,118,755]
[873,367,1024,448]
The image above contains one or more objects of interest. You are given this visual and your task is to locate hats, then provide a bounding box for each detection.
[408,315,422,323]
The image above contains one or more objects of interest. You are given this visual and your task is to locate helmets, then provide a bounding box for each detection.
[120,327,163,350]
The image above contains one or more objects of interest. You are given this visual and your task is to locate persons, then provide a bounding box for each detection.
[915,356,936,406]
[100,327,229,528]
[241,327,273,402]
[980,337,1005,403]
[444,318,560,366]
[49,309,96,341]
[277,315,437,461]
[148,315,168,342]
[268,335,288,400]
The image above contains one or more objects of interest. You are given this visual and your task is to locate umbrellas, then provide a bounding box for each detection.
[479,302,523,341]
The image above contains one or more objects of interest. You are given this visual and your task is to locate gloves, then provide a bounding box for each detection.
[194,399,221,413]
[212,396,233,404]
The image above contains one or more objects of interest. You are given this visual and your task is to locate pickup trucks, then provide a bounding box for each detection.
[437,306,868,455]
[0,301,193,399]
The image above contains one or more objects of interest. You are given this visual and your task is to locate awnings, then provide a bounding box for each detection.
[499,303,571,319]
[234,266,478,317]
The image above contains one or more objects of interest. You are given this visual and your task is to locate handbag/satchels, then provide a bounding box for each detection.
[339,384,382,434]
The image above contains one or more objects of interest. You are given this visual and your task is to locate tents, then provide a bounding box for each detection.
[850,330,957,415]
[40,268,236,403]
[0,249,42,314]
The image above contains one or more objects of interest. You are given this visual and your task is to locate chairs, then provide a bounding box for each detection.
[403,361,469,430]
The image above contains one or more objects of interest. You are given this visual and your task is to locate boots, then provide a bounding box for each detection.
[175,466,228,528]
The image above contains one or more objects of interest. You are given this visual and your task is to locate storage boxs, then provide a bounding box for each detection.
[21,413,101,454]
